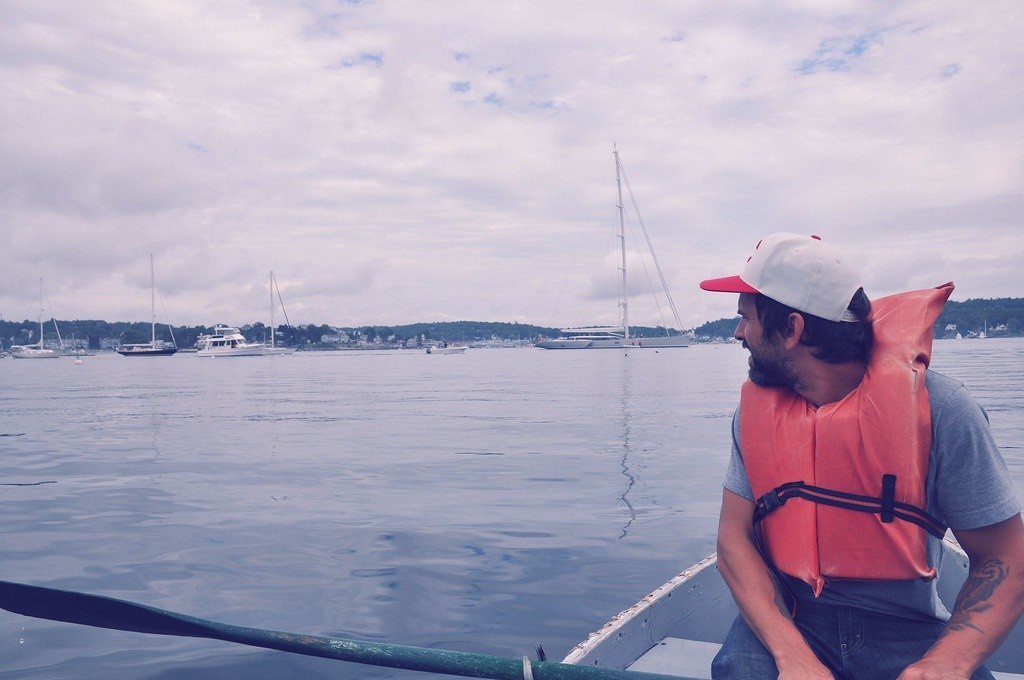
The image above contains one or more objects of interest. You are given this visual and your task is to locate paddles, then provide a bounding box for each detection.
[0,577,699,680]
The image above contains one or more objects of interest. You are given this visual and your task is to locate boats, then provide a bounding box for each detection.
[192,324,266,357]
[425,342,467,354]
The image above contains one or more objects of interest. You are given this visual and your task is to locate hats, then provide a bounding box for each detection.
[701,233,863,322]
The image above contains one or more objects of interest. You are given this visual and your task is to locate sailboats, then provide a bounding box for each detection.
[0,276,96,358]
[114,253,181,357]
[261,270,298,356]
[533,140,690,350]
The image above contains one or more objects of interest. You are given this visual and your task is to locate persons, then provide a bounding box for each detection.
[700,232,1024,680]
